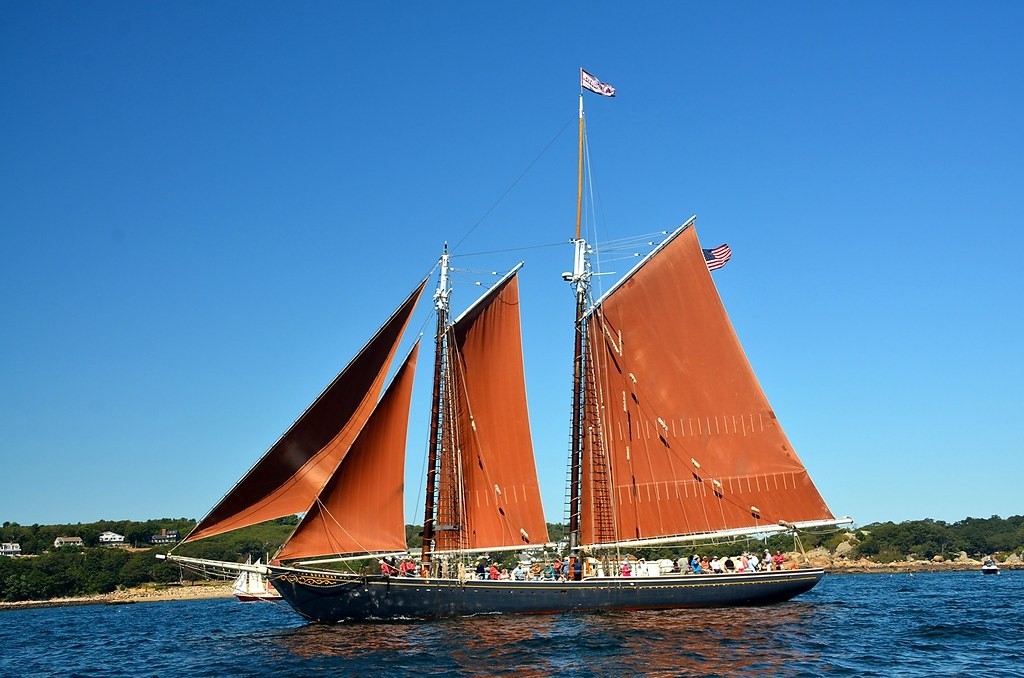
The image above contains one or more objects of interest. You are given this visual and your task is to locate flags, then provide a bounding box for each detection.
[701,243,734,273]
[581,68,620,100]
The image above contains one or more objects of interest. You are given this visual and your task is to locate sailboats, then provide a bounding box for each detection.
[151,63,853,604]
[230,552,282,602]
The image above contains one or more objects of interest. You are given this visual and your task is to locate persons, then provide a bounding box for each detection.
[388,557,397,576]
[415,545,763,587]
[406,557,416,578]
[380,556,390,577]
[763,549,773,572]
[774,550,784,571]
[399,558,408,578]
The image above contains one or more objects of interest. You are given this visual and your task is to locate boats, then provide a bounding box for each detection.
[981,555,1000,575]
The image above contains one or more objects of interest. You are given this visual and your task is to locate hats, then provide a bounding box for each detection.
[693,554,700,559]
[704,556,707,558]
[713,556,718,558]
[764,549,769,552]
[625,553,631,557]
[474,561,480,566]
